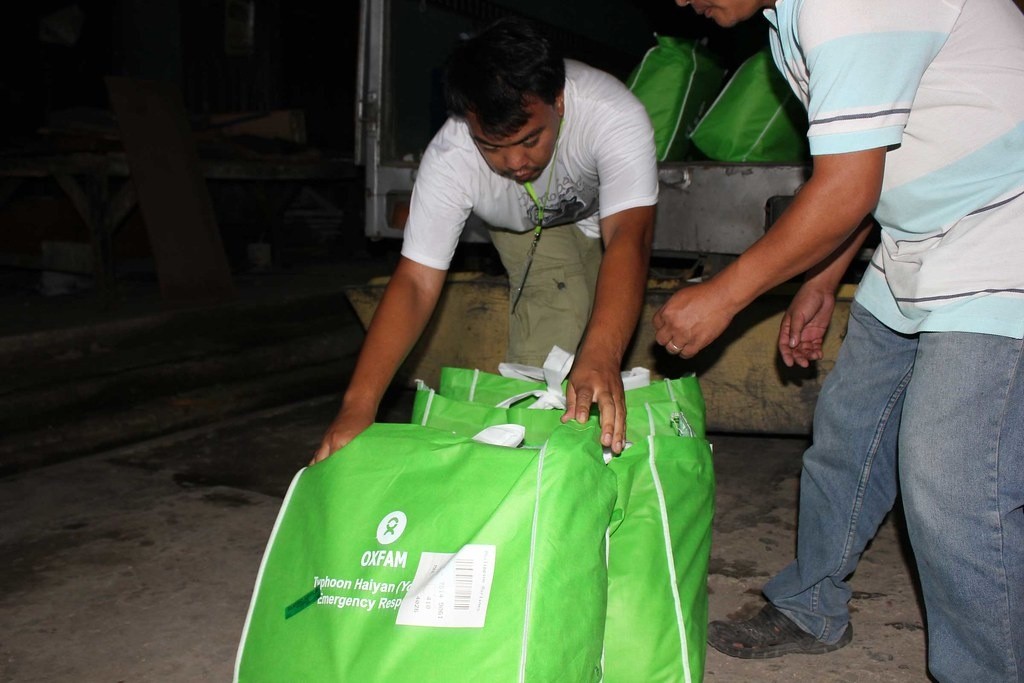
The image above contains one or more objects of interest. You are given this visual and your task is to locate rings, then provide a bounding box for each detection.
[671,339,684,351]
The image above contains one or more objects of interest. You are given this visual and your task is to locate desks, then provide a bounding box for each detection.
[0,152,321,302]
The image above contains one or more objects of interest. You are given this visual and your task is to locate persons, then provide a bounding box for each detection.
[308,12,660,467]
[651,0,1024,683]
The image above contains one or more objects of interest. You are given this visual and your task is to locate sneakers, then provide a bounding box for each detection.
[706,600,853,659]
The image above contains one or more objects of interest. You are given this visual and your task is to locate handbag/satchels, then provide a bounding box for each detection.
[232,344,716,683]
[625,34,730,162]
[688,47,810,163]
